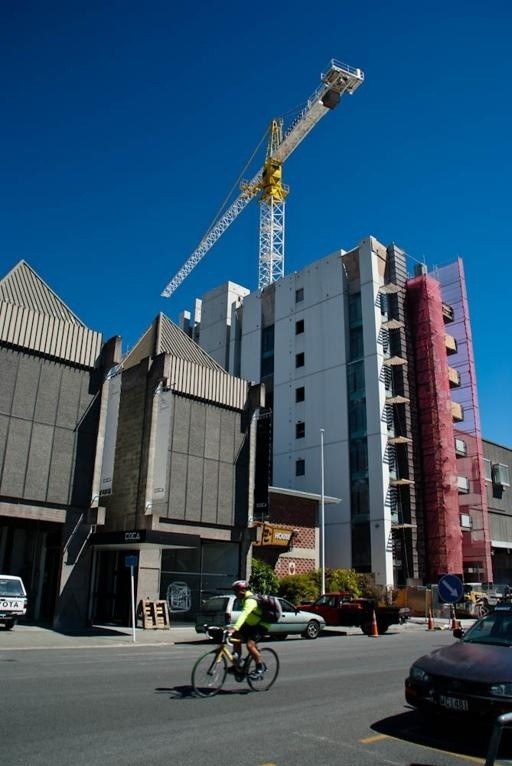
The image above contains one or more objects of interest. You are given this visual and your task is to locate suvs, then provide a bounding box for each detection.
[1,574,29,630]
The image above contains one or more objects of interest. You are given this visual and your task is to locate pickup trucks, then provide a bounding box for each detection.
[296,592,410,635]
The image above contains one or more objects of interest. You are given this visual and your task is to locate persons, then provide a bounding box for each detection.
[223,579,267,679]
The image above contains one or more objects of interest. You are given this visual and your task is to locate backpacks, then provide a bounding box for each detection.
[243,592,281,624]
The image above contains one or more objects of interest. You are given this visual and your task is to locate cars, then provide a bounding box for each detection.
[406,601,512,743]
[195,594,326,643]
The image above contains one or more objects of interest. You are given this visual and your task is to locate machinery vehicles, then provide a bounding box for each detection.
[455,591,498,618]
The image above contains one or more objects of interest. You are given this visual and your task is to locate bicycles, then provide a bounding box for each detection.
[191,623,279,698]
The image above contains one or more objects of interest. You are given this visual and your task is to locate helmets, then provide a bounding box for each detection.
[231,580,250,590]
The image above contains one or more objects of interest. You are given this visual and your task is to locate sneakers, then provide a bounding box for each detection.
[223,665,240,674]
[252,662,267,677]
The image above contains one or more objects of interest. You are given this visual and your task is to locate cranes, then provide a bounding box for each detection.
[160,55,364,299]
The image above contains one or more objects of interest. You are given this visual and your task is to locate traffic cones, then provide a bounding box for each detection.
[450,606,460,631]
[369,607,379,638]
[427,607,436,631]
